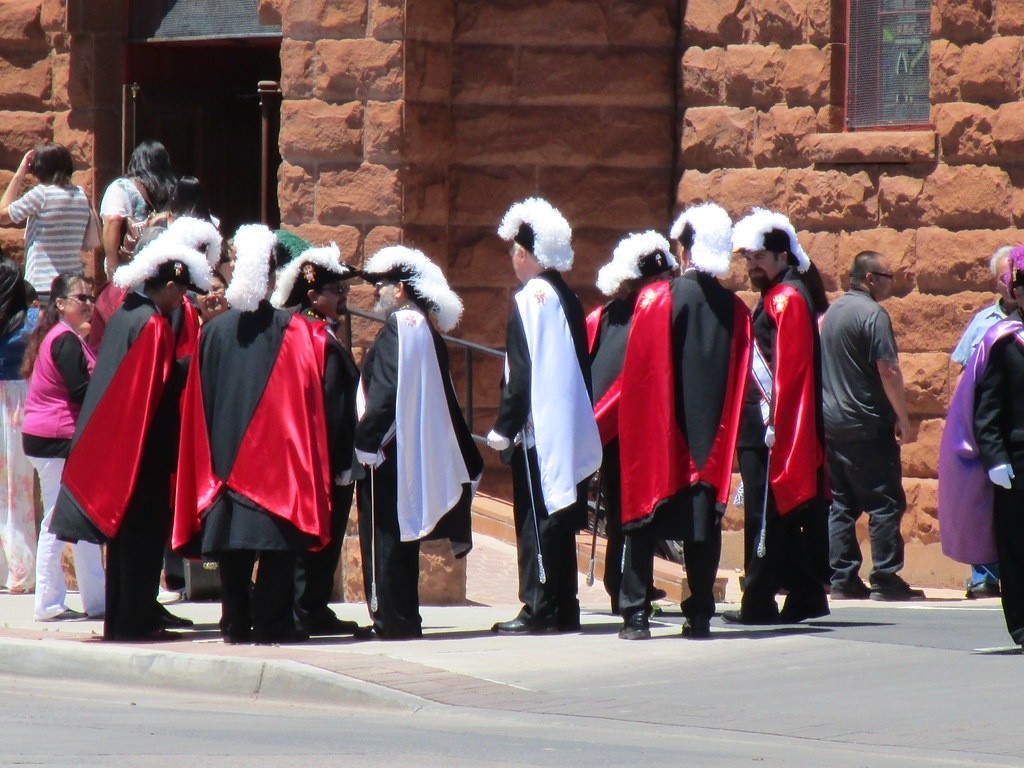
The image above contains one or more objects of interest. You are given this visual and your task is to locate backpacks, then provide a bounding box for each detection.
[118,176,168,264]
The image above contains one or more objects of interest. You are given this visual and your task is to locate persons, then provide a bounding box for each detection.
[951,246,1018,600]
[22,273,106,624]
[0,247,47,594]
[150,175,222,230]
[0,142,89,314]
[354,245,483,641]
[486,197,602,636]
[98,140,177,281]
[821,250,927,602]
[48,216,363,643]
[939,245,1024,654]
[586,202,829,641]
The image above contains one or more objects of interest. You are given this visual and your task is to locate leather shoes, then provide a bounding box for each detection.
[619,609,652,640]
[965,582,1001,598]
[721,603,830,626]
[491,616,582,636]
[682,616,711,638]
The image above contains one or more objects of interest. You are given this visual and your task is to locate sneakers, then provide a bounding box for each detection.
[831,577,870,599]
[870,575,927,602]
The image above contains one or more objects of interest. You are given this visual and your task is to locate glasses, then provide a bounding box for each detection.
[870,271,895,283]
[64,294,96,303]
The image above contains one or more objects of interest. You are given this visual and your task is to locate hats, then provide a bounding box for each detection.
[731,207,812,274]
[359,245,465,333]
[596,229,680,297]
[223,223,278,313]
[112,216,223,295]
[270,240,363,311]
[498,197,575,272]
[669,202,733,278]
[1004,245,1024,300]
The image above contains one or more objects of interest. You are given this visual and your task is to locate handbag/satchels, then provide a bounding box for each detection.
[80,187,103,252]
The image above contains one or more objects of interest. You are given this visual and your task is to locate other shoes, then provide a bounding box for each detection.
[39,583,426,645]
[611,585,667,613]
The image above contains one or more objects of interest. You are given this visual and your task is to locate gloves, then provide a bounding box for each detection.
[486,429,510,451]
[764,425,776,449]
[988,463,1015,489]
[354,448,378,465]
[335,469,352,486]
